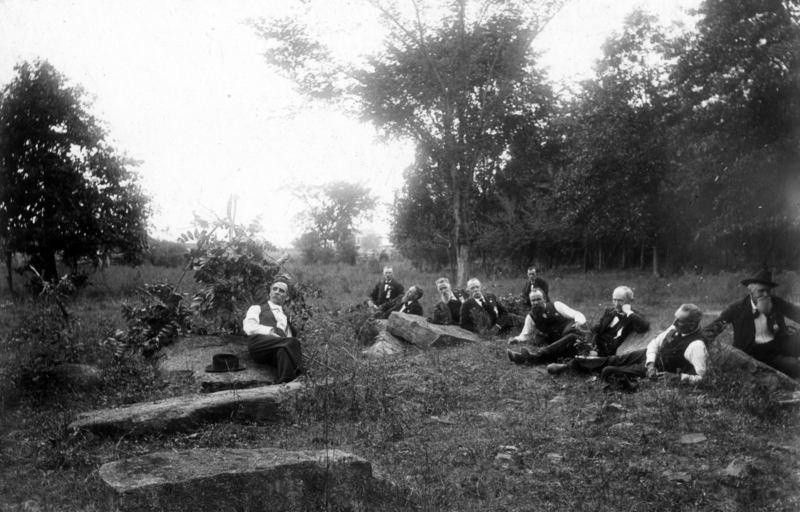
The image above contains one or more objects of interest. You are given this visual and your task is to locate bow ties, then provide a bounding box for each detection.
[615,312,624,319]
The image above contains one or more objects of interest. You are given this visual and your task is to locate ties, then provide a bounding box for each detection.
[479,299,485,305]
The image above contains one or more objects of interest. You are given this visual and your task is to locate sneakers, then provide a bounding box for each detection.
[547,363,569,372]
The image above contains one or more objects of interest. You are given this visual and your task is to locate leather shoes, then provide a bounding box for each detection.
[507,348,538,364]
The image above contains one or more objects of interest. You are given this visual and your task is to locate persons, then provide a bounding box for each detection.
[427,277,470,325]
[521,266,549,306]
[505,286,587,344]
[368,264,403,311]
[244,280,303,382]
[506,285,650,365]
[460,279,526,338]
[547,302,709,389]
[373,285,423,319]
[703,270,800,378]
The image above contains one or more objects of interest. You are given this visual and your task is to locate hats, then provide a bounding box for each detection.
[740,271,778,287]
[206,354,246,372]
